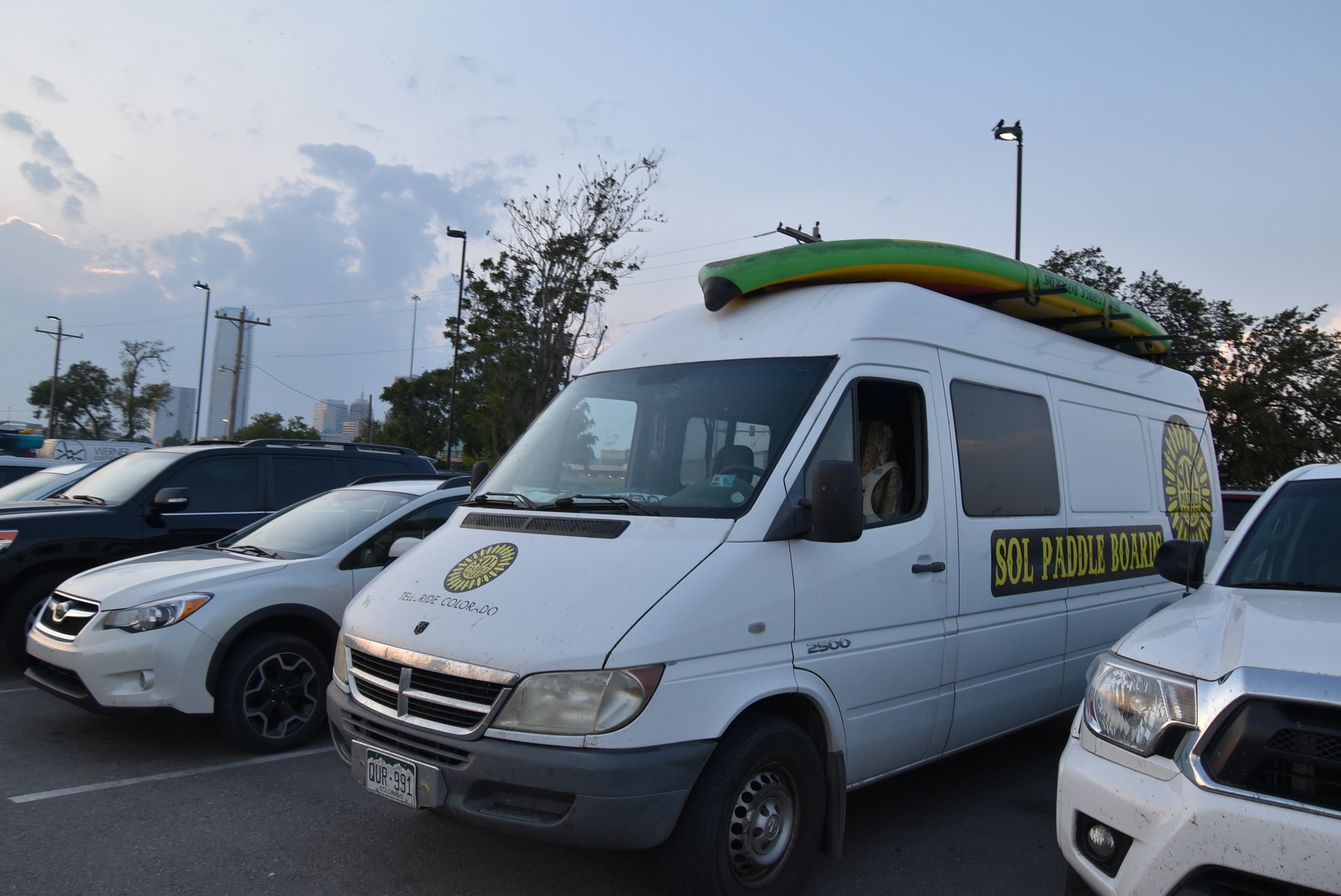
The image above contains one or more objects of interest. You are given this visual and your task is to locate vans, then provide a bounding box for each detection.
[325,282,1224,896]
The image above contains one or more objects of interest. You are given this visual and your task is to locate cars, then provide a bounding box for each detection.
[23,472,573,756]
[0,420,160,506]
[1057,462,1340,896]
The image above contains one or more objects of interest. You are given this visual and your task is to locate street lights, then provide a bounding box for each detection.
[994,126,1024,262]
[445,229,468,468]
[46,315,62,438]
[193,284,211,442]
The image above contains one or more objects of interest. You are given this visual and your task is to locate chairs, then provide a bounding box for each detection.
[858,416,906,520]
[713,444,755,485]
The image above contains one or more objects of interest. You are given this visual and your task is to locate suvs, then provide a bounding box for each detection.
[1220,485,1270,545]
[1,437,442,669]
